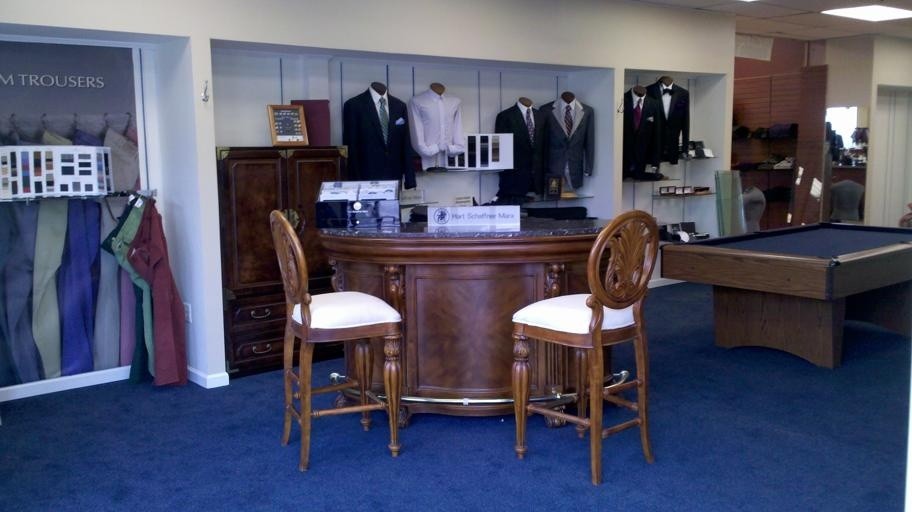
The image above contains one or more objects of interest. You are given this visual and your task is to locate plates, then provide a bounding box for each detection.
[214,142,350,379]
[652,148,717,248]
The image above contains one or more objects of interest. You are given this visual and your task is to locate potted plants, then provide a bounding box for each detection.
[267,101,311,148]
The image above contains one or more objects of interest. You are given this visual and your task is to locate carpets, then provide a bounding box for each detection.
[565,105,572,138]
[633,98,642,130]
[526,108,534,148]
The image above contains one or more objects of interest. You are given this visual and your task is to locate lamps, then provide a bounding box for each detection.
[264,208,403,474]
[510,209,664,487]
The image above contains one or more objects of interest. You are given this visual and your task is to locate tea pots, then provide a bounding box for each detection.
[820,103,871,224]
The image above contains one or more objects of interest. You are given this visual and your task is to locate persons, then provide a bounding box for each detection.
[624,85,666,168]
[539,91,594,187]
[343,82,417,190]
[408,83,466,167]
[495,96,551,196]
[647,76,690,164]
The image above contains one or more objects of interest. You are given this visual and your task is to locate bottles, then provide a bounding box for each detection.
[379,97,390,146]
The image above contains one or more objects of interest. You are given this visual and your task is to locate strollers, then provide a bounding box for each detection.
[662,88,673,96]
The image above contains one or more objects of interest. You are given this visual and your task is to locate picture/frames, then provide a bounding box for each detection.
[660,219,911,373]
[312,217,618,431]
